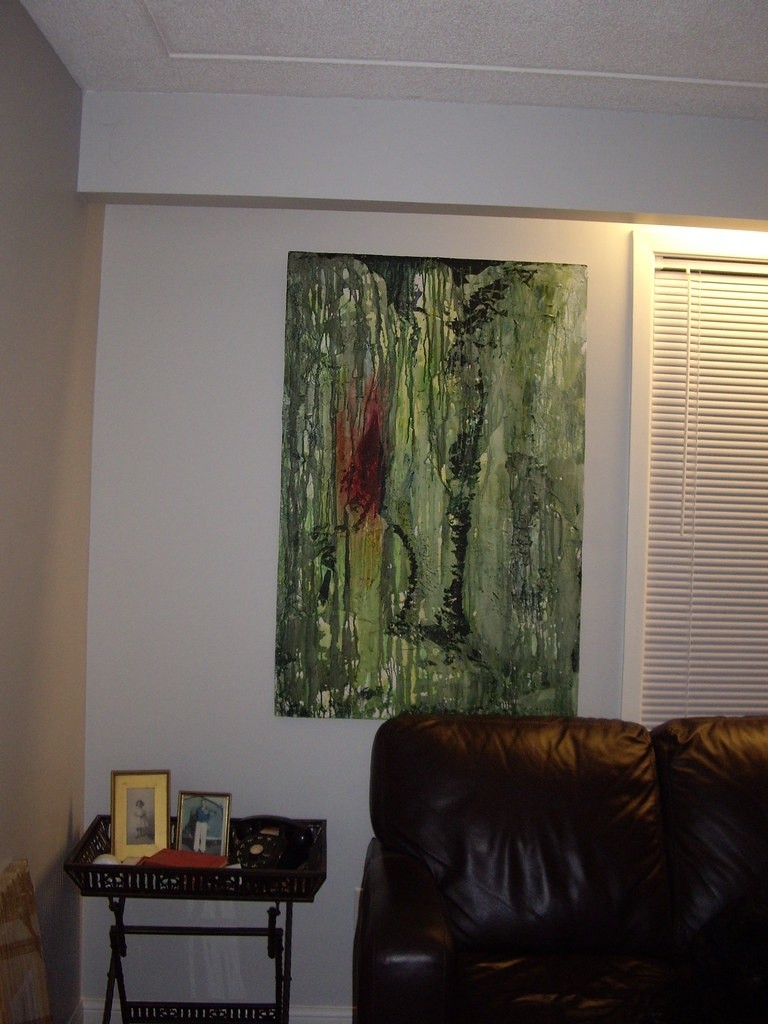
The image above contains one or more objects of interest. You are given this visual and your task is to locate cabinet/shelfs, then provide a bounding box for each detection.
[63,813,327,1024]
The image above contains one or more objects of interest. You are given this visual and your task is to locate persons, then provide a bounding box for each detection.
[135,800,148,838]
[194,799,210,853]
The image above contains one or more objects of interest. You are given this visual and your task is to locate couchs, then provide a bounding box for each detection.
[352,709,768,1024]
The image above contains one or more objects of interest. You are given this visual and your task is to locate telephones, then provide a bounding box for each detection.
[229,813,315,882]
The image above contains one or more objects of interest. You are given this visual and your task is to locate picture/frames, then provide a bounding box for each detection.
[176,790,232,856]
[111,769,171,861]
